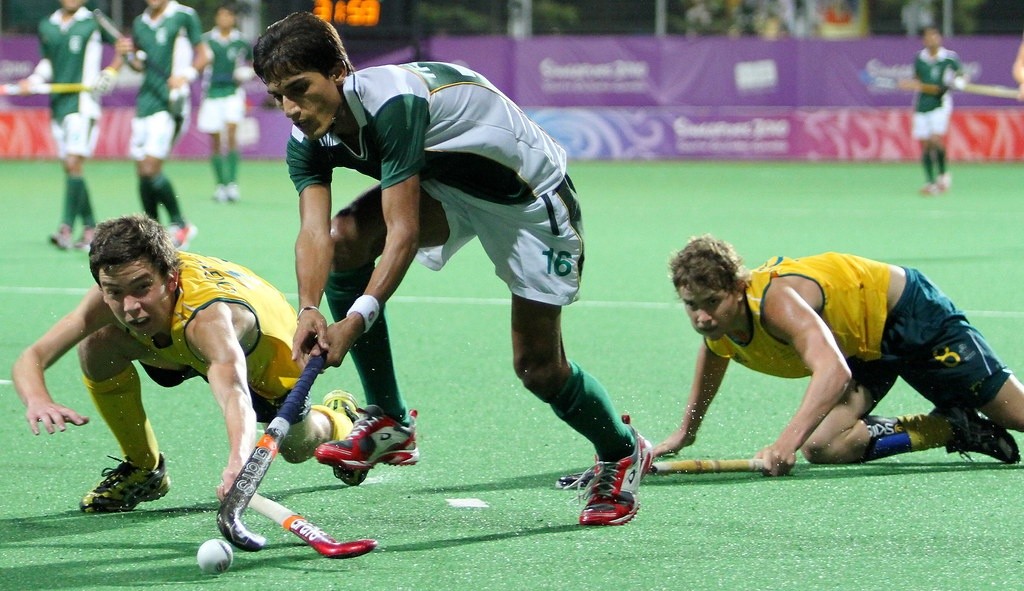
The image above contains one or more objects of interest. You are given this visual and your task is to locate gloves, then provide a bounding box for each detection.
[92,66,117,97]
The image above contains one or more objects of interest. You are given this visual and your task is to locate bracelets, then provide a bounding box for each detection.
[346,295,381,334]
[297,305,320,323]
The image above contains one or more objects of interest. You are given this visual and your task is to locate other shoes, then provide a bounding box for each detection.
[74,225,96,252]
[214,183,240,203]
[170,222,199,252]
[49,223,73,249]
[922,182,938,194]
[938,172,951,191]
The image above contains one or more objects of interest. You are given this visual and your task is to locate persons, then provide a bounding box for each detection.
[10,217,368,517]
[251,12,651,526]
[910,26,969,195]
[650,236,1024,478]
[10,1,124,251]
[114,0,214,251]
[198,3,260,205]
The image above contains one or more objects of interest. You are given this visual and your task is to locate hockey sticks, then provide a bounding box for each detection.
[865,58,940,94]
[216,348,326,552]
[248,492,378,559]
[93,8,184,141]
[558,453,770,493]
[956,72,1024,98]
[0,82,92,95]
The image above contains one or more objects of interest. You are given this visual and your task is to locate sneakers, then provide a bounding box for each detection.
[929,405,1021,465]
[79,452,170,514]
[563,414,653,526]
[322,390,370,486]
[313,404,420,469]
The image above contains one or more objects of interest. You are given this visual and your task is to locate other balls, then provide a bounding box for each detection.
[197,539,233,574]
